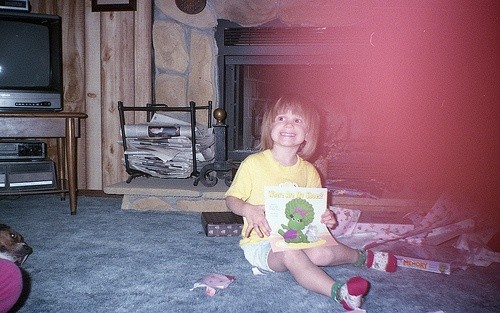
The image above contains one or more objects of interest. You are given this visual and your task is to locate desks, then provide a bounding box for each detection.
[0,111,90,215]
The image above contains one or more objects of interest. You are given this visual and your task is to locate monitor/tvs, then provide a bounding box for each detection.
[0,11,63,113]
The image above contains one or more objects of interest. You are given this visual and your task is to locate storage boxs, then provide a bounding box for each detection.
[200,210,244,238]
[367,240,466,274]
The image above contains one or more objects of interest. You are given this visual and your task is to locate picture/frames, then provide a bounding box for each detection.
[90,0,138,12]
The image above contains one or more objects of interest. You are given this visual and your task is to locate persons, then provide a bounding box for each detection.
[223,94,398,311]
[0,258,22,312]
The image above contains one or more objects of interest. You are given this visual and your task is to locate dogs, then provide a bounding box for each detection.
[0,223,33,267]
